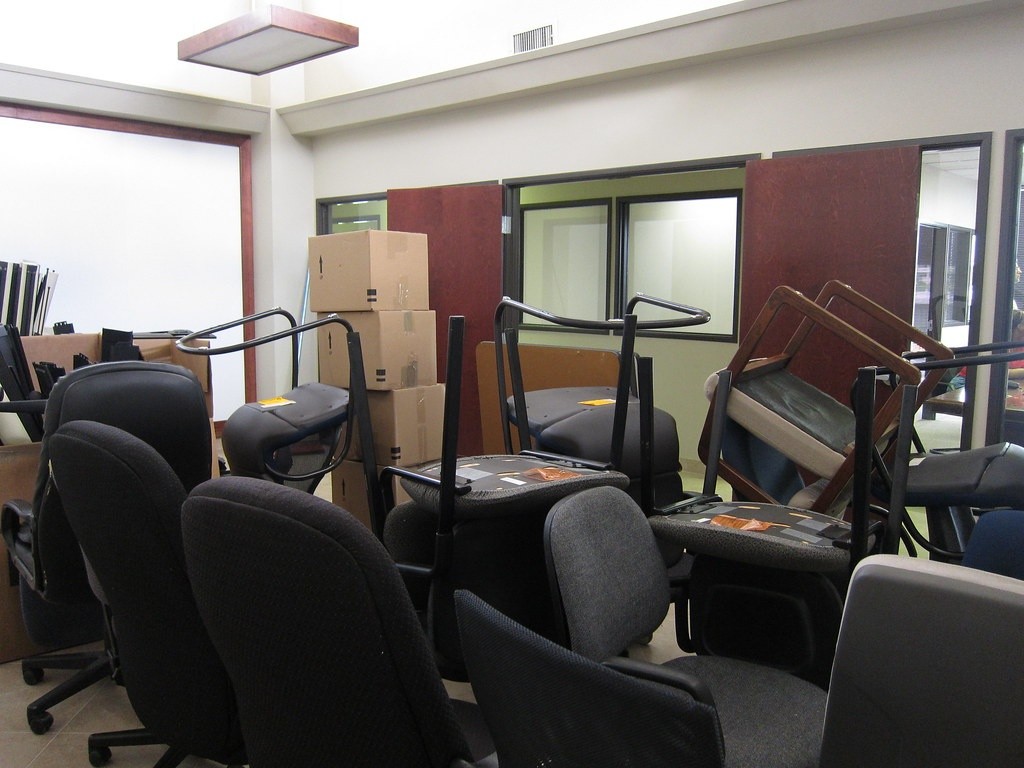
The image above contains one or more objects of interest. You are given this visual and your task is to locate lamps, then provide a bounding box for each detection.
[179,4,359,76]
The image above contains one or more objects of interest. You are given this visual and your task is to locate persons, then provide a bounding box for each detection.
[946,310,1024,393]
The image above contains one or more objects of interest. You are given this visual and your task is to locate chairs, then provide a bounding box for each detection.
[0,282,1024,768]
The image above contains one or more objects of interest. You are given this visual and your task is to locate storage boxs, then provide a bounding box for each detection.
[307,228,445,527]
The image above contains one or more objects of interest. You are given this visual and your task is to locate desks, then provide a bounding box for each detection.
[922,377,1024,437]
[476,340,622,457]
